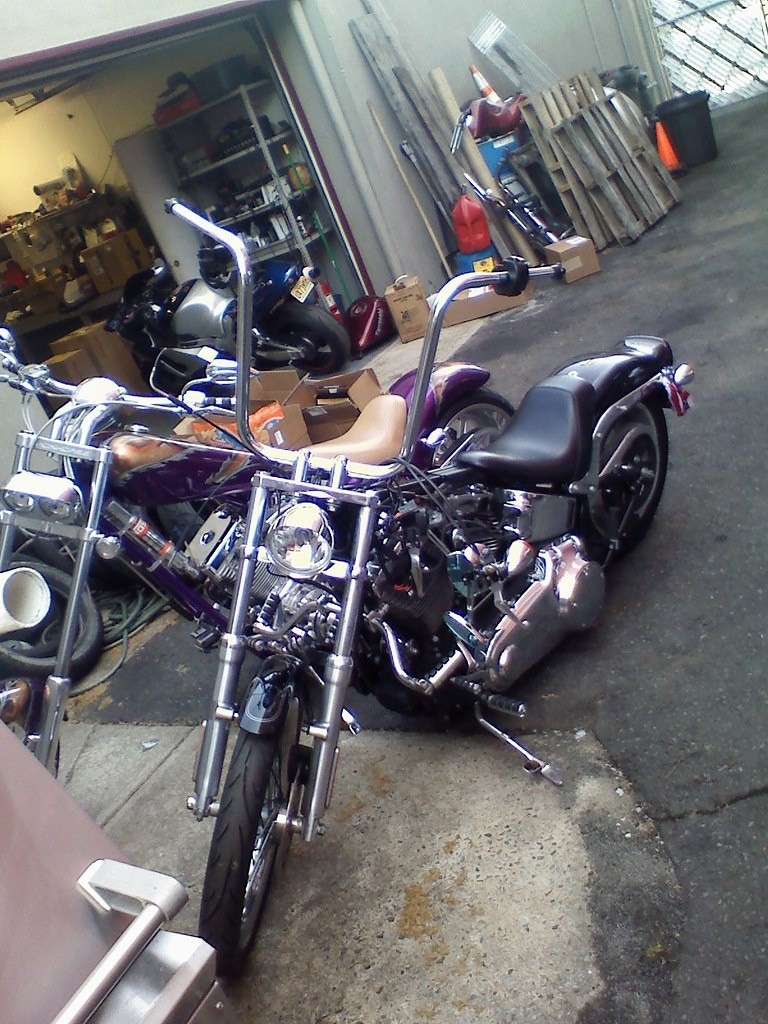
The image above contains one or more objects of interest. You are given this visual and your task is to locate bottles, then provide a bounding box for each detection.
[236,221,277,254]
[4,211,125,305]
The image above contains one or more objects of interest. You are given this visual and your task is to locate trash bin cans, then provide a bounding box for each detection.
[655,91,719,169]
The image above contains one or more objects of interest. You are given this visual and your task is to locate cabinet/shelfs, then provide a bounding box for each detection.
[0,76,363,364]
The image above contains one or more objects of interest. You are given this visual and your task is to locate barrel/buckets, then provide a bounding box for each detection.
[452,184,491,254]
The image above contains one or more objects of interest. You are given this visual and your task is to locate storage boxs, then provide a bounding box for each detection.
[384,275,533,344]
[191,53,246,104]
[25,283,57,315]
[544,236,600,283]
[173,367,382,453]
[81,230,149,293]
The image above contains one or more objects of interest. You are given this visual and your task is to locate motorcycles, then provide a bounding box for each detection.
[0,328,515,777]
[100,254,352,399]
[163,199,695,982]
